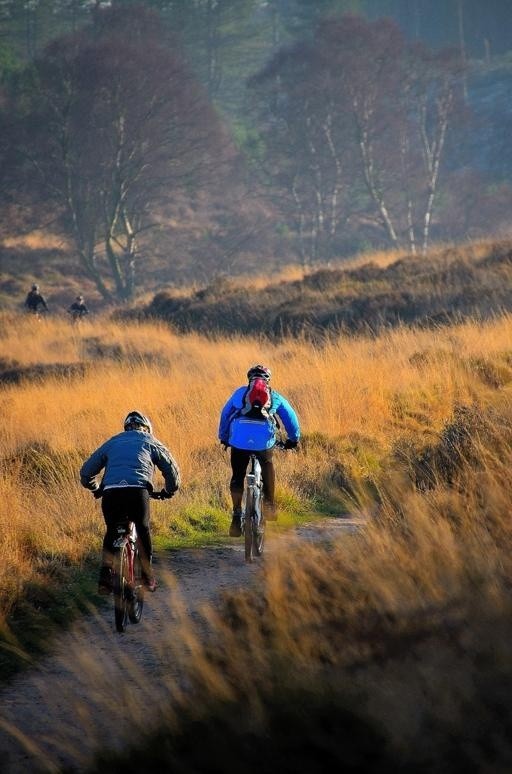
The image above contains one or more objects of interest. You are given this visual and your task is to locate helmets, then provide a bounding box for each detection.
[124,412,152,433]
[247,365,271,382]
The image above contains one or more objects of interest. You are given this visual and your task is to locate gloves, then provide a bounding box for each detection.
[284,440,298,450]
[160,489,172,500]
[92,488,101,499]
[221,440,230,447]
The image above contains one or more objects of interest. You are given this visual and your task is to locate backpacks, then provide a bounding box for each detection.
[229,378,272,422]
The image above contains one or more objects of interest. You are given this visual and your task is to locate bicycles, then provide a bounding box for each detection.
[95,492,161,632]
[224,440,284,564]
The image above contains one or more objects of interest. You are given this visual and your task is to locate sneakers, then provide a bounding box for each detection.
[98,582,111,595]
[263,501,277,521]
[230,516,241,537]
[143,578,160,592]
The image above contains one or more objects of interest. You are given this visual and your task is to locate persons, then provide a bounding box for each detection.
[24,283,49,314]
[66,294,89,320]
[79,409,182,596]
[218,363,301,538]
